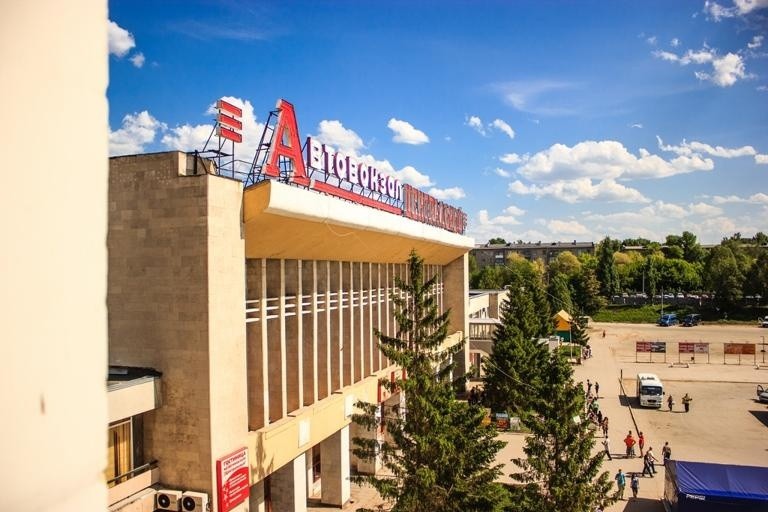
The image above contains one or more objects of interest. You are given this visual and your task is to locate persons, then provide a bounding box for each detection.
[629,431,635,457]
[586,392,609,438]
[602,436,612,460]
[661,441,670,454]
[667,394,673,411]
[613,469,626,501]
[664,447,671,466]
[643,452,653,478]
[629,472,639,499]
[648,447,659,473]
[624,436,636,458]
[682,393,692,413]
[586,379,592,393]
[595,382,600,398]
[638,432,644,457]
[466,385,487,405]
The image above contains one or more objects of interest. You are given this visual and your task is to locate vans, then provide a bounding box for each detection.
[659,312,677,328]
[683,313,702,327]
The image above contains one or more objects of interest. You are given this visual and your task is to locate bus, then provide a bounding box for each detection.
[636,372,666,410]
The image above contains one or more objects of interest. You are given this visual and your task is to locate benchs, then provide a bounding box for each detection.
[671,362,690,368]
[757,364,768,370]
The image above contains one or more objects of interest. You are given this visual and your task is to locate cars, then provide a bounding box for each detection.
[612,292,761,299]
[758,315,768,328]
[755,383,768,403]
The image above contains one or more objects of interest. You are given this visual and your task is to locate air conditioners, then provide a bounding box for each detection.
[157,490,182,511]
[182,491,208,512]
